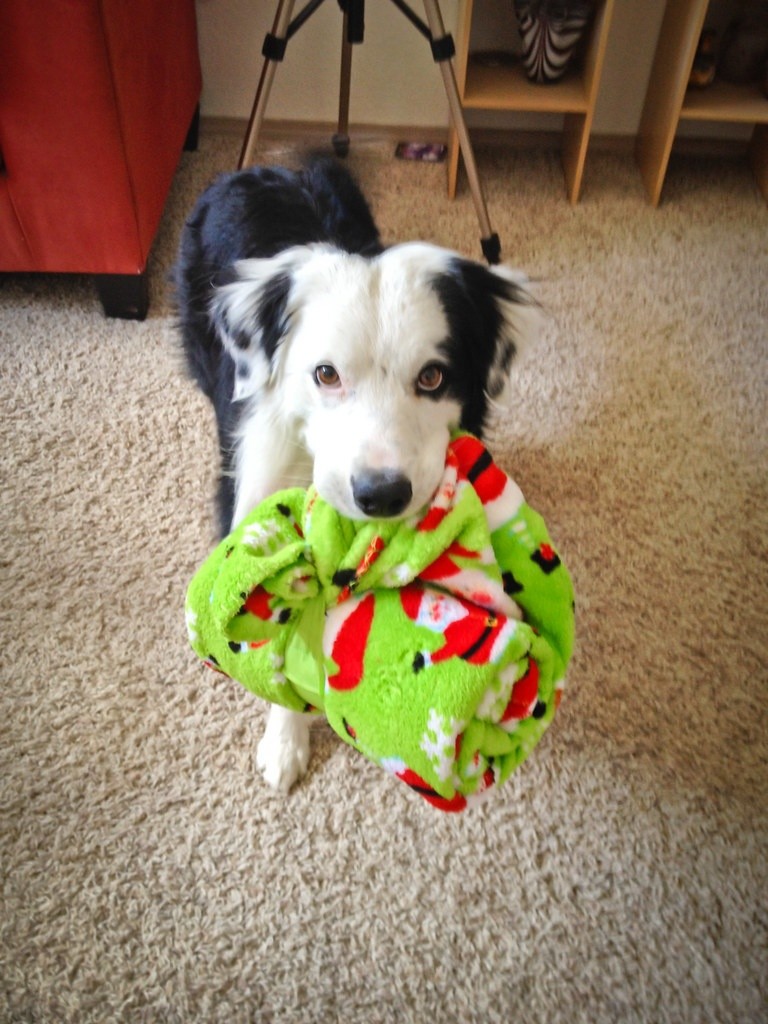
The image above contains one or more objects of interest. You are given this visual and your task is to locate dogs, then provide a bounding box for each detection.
[170,151,547,795]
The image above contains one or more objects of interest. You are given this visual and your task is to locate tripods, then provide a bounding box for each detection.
[235,0,505,265]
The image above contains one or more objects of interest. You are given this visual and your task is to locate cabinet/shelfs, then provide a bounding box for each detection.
[447,0,768,209]
[0,0,203,321]
